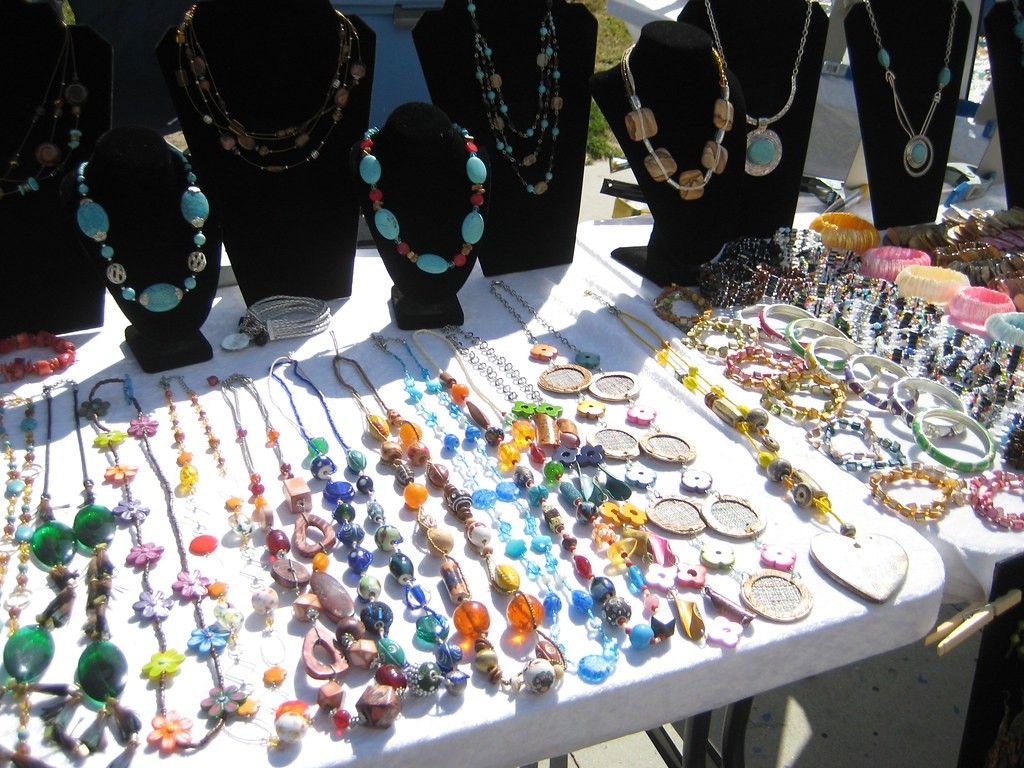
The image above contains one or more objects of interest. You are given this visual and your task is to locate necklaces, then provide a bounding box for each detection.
[1011,0,1024,66]
[79,374,247,752]
[467,0,563,195]
[360,123,487,274]
[705,0,812,177]
[176,5,363,173]
[77,144,209,313]
[0,0,87,196]
[0,317,757,768]
[862,0,954,178]
[488,277,816,625]
[583,284,907,602]
[621,42,734,200]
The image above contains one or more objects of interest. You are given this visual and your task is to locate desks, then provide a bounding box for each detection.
[0,214,1024,768]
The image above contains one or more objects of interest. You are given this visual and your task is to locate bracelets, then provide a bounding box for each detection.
[845,354,920,412]
[651,208,1024,534]
[0,330,75,383]
[786,317,853,368]
[223,294,331,350]
